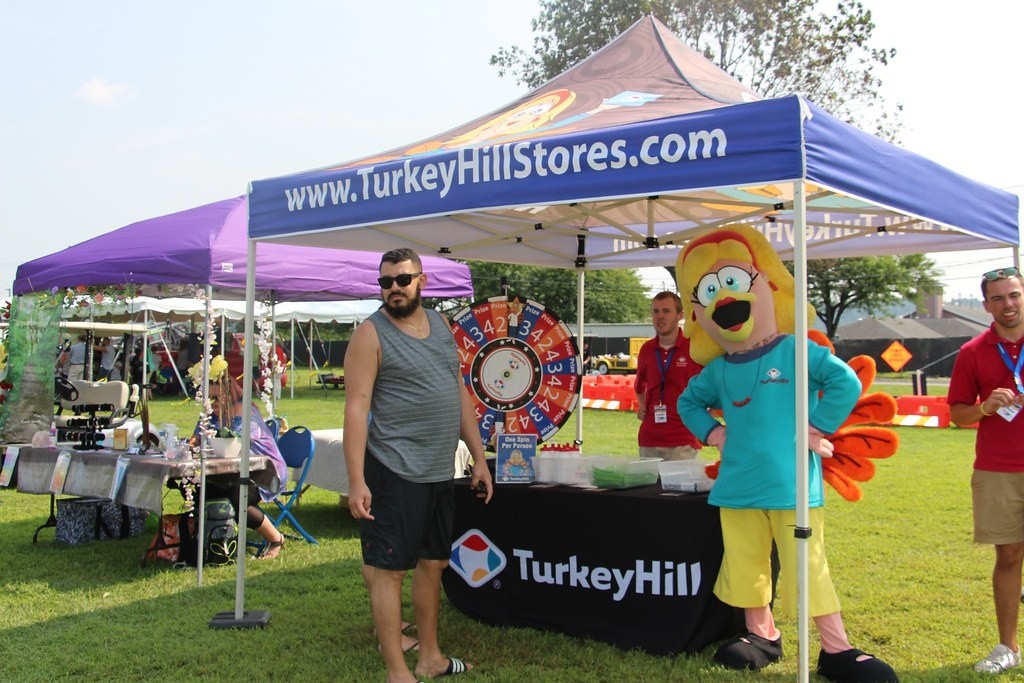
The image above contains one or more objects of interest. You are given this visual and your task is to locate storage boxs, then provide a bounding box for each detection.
[583,455,663,490]
[52,496,145,544]
[529,453,613,486]
[658,456,720,493]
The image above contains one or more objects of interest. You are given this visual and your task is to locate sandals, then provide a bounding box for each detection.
[258,533,285,560]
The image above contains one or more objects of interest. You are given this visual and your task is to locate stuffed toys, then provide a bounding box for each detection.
[676,223,899,683]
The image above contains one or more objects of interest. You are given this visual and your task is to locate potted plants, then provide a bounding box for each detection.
[209,426,243,459]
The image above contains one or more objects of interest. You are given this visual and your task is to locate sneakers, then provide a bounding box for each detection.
[974,644,1022,676]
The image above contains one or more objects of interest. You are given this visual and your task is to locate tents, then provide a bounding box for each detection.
[234,15,1024,683]
[0,294,382,399]
[12,194,474,588]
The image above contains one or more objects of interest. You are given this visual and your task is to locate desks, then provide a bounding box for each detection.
[445,471,780,666]
[316,380,345,391]
[0,438,272,571]
[290,428,472,509]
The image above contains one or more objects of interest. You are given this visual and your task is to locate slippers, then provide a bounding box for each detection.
[414,657,474,680]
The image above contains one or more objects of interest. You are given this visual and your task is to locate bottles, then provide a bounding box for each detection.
[561,445,570,483]
[556,444,563,482]
[49,420,57,450]
[570,445,580,484]
[549,443,556,482]
[540,444,552,483]
[566,443,572,483]
[158,432,166,459]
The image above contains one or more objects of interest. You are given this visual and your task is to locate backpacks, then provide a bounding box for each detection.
[178,499,238,568]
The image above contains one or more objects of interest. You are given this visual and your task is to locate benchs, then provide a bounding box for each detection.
[53,374,140,428]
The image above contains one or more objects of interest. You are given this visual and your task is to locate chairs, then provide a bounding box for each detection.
[263,418,280,442]
[249,424,317,557]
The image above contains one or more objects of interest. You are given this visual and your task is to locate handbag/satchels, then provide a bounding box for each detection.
[147,513,195,562]
[56,497,146,547]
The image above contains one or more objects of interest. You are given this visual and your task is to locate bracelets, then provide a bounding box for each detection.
[981,402,994,416]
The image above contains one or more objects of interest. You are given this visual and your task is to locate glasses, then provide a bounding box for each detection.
[982,266,1022,283]
[208,395,224,402]
[378,272,422,289]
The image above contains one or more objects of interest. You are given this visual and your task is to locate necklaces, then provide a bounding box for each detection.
[723,348,763,406]
[400,318,423,336]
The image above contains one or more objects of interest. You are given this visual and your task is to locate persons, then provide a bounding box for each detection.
[583,341,591,375]
[132,348,143,401]
[147,338,155,400]
[151,345,162,384]
[94,337,116,380]
[634,291,705,461]
[191,372,289,559]
[343,249,493,683]
[947,267,1024,674]
[177,338,189,374]
[68,335,86,380]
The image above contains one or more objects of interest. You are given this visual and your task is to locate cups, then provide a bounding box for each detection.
[172,444,190,463]
